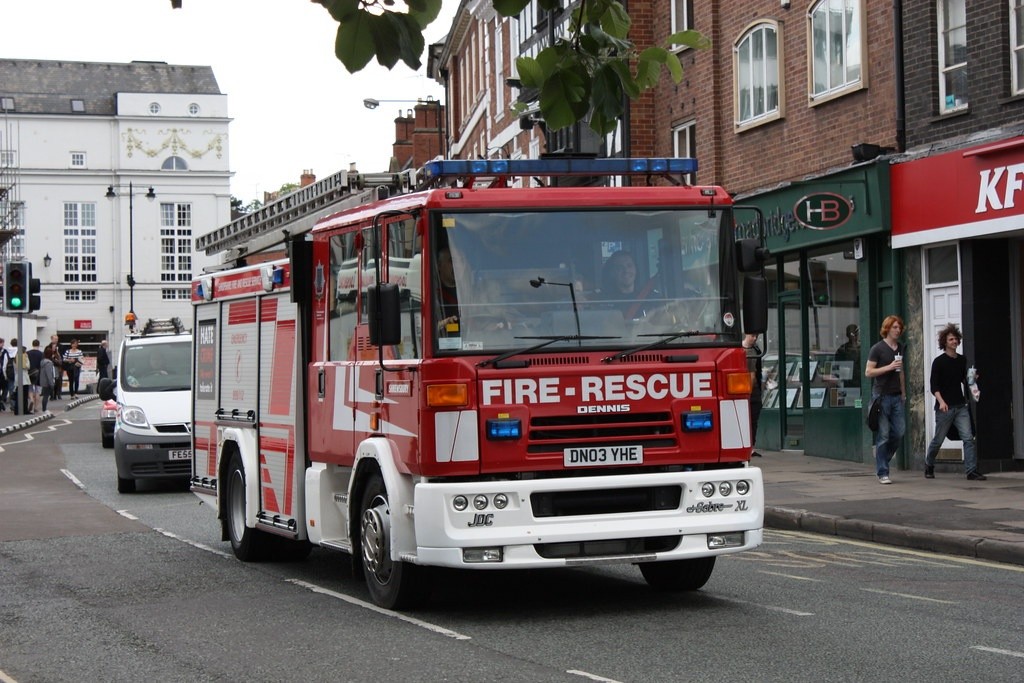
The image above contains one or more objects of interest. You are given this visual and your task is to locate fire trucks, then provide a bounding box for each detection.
[189,151,770,612]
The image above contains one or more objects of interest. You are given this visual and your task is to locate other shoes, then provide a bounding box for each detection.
[71,397,74,400]
[58,396,61,399]
[925,462,934,478]
[751,452,761,457]
[42,410,50,414]
[75,395,78,399]
[876,473,891,484]
[967,471,986,480]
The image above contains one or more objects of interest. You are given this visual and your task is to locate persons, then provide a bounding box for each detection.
[924,323,987,481]
[44,335,58,359]
[742,330,763,457]
[40,347,55,413]
[0,339,11,412]
[8,339,18,410]
[438,246,475,335]
[63,339,84,399]
[865,315,906,484]
[14,346,31,415]
[27,340,44,412]
[835,324,861,391]
[138,352,174,379]
[97,340,110,394]
[600,250,660,317]
[48,343,62,401]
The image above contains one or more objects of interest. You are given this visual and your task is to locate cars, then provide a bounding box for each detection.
[99,379,115,449]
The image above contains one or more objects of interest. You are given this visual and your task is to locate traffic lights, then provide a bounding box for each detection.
[3,261,28,313]
[28,262,41,313]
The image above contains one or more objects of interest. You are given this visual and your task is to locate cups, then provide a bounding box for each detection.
[894,355,903,371]
[968,368,977,385]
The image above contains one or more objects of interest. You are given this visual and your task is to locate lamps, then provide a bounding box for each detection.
[44,252,52,268]
[851,143,896,161]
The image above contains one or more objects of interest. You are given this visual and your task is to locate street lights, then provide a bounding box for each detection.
[104,180,155,338]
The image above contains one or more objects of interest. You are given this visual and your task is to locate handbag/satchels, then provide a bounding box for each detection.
[947,398,976,440]
[868,399,881,431]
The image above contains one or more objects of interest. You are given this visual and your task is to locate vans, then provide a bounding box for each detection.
[99,331,191,494]
